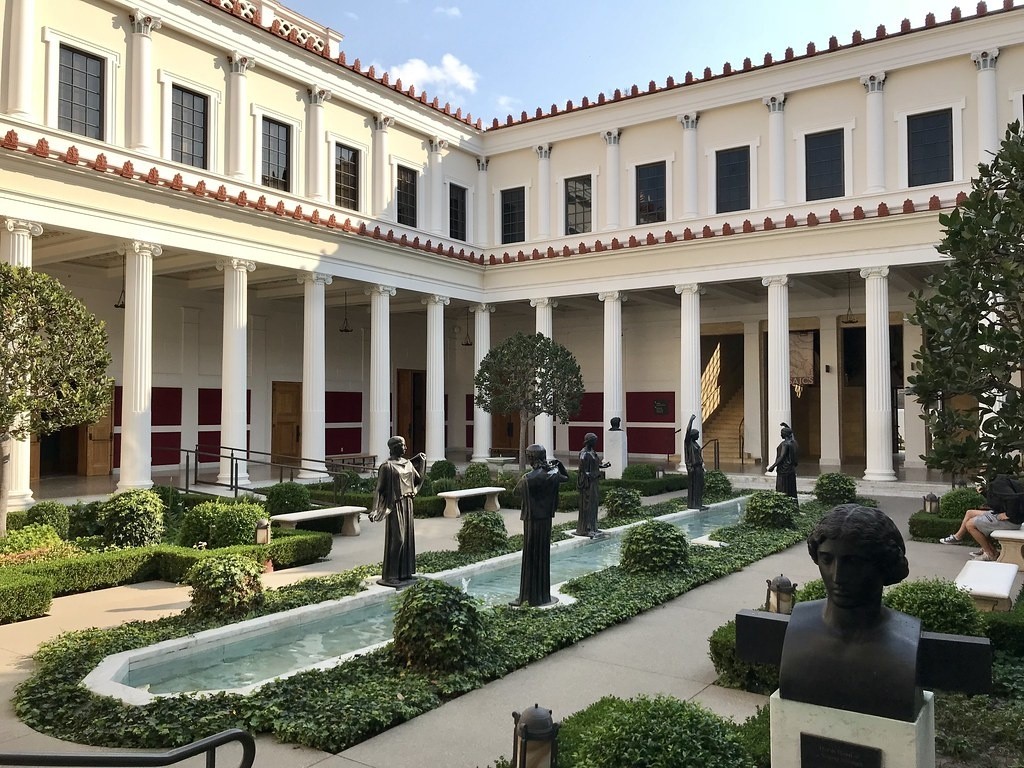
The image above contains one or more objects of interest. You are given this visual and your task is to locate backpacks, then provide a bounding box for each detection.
[988,476,1024,524]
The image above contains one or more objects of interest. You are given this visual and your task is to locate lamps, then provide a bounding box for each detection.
[511,703,561,768]
[922,491,940,514]
[656,466,665,477]
[765,574,798,615]
[255,520,271,544]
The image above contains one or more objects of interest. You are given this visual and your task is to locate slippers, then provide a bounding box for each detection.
[975,552,997,561]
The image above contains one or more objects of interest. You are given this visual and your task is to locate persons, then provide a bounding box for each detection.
[609,417,624,431]
[368,436,428,588]
[684,414,710,511]
[508,444,570,607]
[571,433,611,537]
[778,505,925,723]
[768,422,799,508]
[940,455,1024,561]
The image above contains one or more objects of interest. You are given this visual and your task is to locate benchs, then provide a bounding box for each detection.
[952,560,1018,613]
[991,522,1023,570]
[437,486,506,518]
[271,506,367,536]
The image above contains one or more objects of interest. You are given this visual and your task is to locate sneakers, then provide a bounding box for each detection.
[939,534,963,545]
[969,548,985,557]
